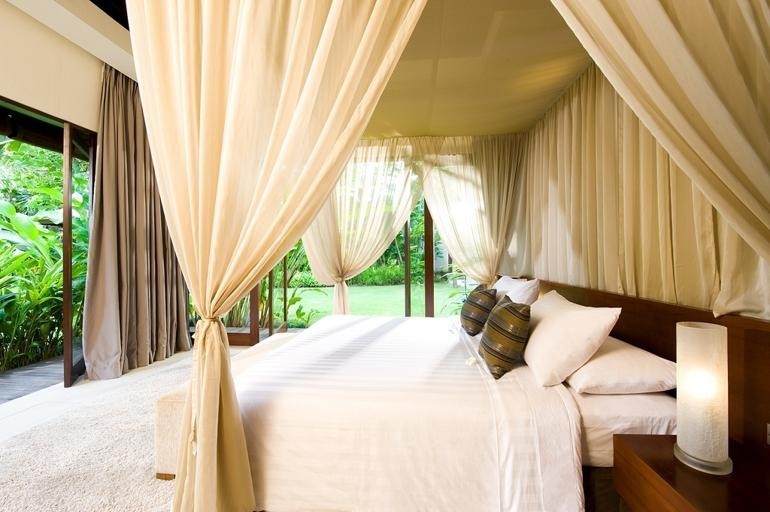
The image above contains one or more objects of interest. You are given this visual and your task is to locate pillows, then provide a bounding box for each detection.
[477,294,532,381]
[520,288,622,387]
[505,278,540,306]
[492,275,528,301]
[460,283,497,336]
[566,335,676,396]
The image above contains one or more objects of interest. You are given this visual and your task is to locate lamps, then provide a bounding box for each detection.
[674,320,736,478]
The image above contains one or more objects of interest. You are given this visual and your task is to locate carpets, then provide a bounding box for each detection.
[0,343,251,512]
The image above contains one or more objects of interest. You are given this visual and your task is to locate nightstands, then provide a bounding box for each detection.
[611,433,769,511]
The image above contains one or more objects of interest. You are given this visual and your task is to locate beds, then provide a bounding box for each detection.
[156,273,735,511]
